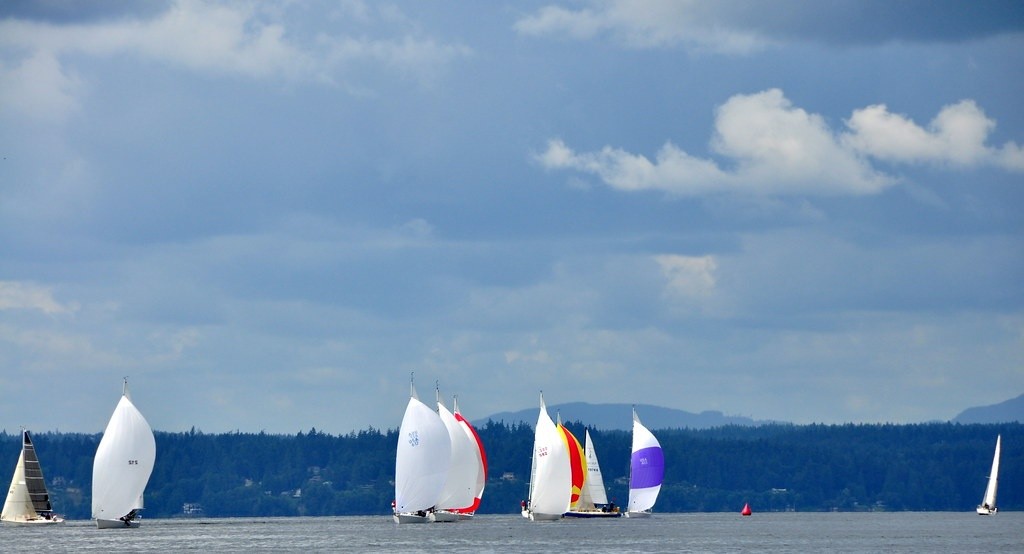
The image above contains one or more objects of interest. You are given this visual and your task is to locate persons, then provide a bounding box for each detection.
[602,500,615,512]
[521,499,526,510]
[985,503,989,509]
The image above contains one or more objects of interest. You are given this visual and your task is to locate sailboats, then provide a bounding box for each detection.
[91,377,156,530]
[976,434,1002,516]
[391,371,487,523]
[623,405,665,518]
[521,390,622,521]
[0,426,65,529]
